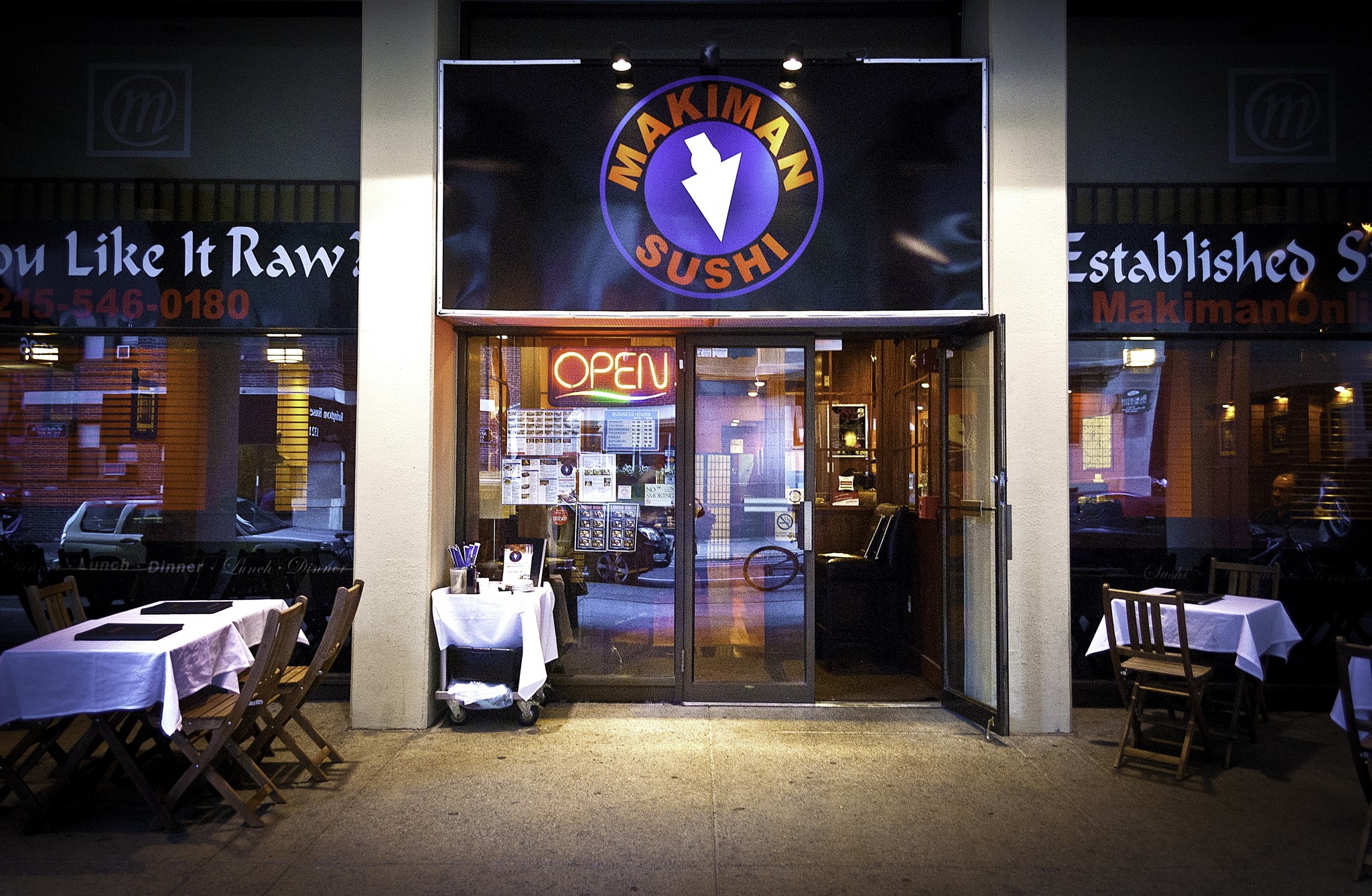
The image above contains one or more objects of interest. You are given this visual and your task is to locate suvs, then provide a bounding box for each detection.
[58,491,355,612]
[478,478,674,584]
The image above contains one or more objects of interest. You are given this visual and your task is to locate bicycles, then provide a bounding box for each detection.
[309,531,355,569]
[742,539,806,590]
[1215,505,1315,581]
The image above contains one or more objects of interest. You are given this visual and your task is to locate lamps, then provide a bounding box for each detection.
[1123,337,1158,369]
[782,39,805,71]
[612,43,632,71]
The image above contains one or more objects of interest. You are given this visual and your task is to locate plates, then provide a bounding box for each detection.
[500,579,534,592]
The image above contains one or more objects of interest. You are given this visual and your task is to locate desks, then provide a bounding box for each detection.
[98,600,309,778]
[1085,585,1302,769]
[0,619,257,843]
[815,555,900,674]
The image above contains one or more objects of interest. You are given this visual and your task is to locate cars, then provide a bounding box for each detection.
[1070,489,1268,575]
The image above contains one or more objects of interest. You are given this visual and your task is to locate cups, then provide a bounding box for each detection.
[450,567,476,595]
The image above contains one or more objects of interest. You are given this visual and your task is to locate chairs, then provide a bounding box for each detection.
[1335,636,1372,881]
[1,540,339,634]
[1101,556,1282,780]
[0,575,365,828]
